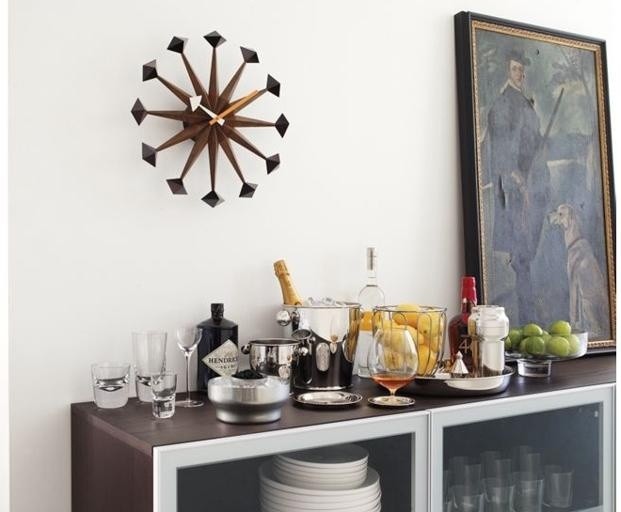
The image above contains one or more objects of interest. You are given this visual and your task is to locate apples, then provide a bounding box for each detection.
[504,319,578,358]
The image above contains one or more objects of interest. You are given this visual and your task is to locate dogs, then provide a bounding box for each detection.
[546,204,611,340]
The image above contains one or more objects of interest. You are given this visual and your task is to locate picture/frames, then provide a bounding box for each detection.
[454,12,616,362]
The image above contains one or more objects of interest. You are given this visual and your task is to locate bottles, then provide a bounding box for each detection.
[448,277,482,378]
[273,260,303,308]
[196,303,239,405]
[358,247,385,378]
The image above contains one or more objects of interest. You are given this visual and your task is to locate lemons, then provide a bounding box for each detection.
[358,304,444,376]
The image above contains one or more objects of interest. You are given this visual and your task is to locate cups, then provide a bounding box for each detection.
[90,332,177,418]
[443,444,575,512]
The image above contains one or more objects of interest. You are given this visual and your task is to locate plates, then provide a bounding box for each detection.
[258,444,382,512]
[368,396,416,407]
[292,391,363,408]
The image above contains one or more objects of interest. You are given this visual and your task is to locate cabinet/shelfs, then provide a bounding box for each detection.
[71,383,616,512]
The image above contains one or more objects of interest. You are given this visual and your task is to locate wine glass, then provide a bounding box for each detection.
[174,328,204,408]
[367,328,419,405]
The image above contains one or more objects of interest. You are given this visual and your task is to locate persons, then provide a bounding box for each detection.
[488,49,570,332]
